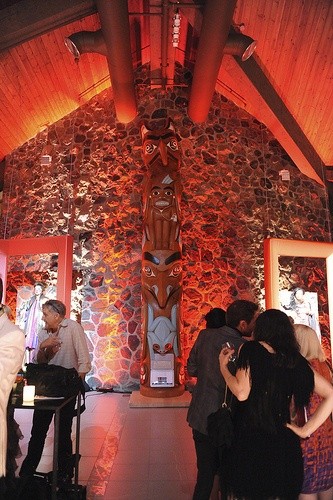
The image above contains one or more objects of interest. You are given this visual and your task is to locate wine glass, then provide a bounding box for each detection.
[221,341,238,368]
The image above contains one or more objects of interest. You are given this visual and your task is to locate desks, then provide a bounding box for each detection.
[9,386,81,500]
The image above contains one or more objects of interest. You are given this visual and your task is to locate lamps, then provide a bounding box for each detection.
[233,23,246,31]
[171,7,182,47]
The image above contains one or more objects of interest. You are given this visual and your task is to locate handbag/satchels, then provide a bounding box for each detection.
[205,403,235,447]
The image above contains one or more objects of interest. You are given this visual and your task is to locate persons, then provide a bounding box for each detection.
[23,282,49,364]
[281,287,313,326]
[0,279,25,481]
[18,301,91,500]
[219,309,333,500]
[186,299,260,500]
[291,324,333,500]
